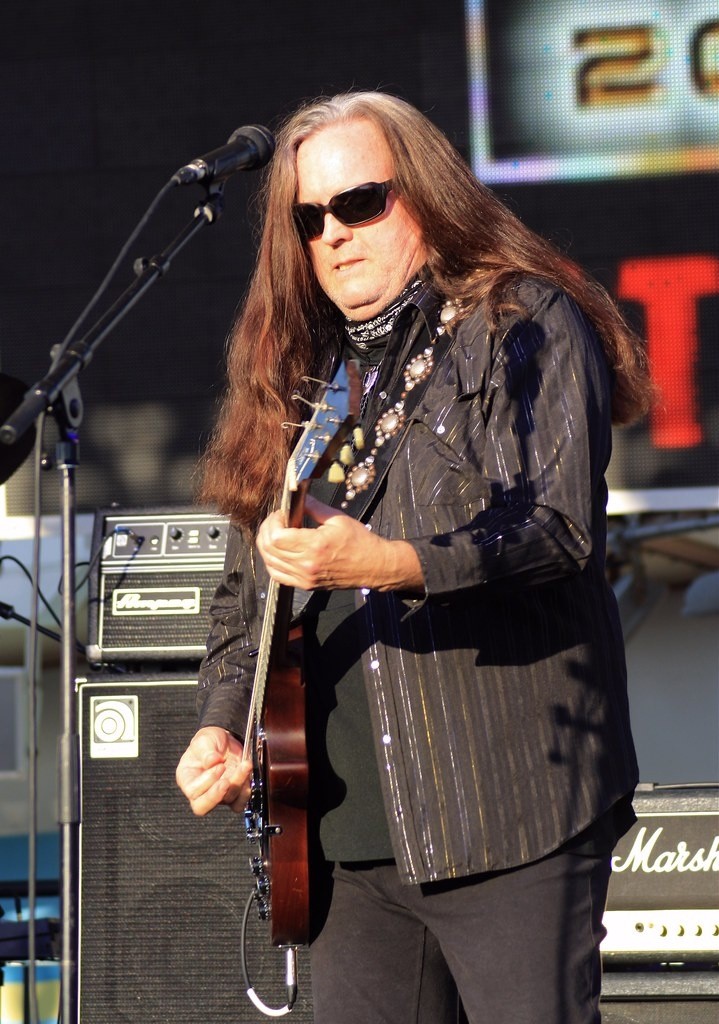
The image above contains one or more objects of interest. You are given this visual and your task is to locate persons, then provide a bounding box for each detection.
[176,86,667,1024]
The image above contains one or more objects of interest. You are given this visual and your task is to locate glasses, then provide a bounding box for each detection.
[291,176,406,240]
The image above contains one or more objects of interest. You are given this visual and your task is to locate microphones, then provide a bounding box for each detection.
[168,118,276,190]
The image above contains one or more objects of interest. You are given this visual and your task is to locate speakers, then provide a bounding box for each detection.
[600,782,719,972]
[72,674,319,1023]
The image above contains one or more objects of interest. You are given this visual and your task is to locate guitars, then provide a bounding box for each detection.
[237,358,365,949]
